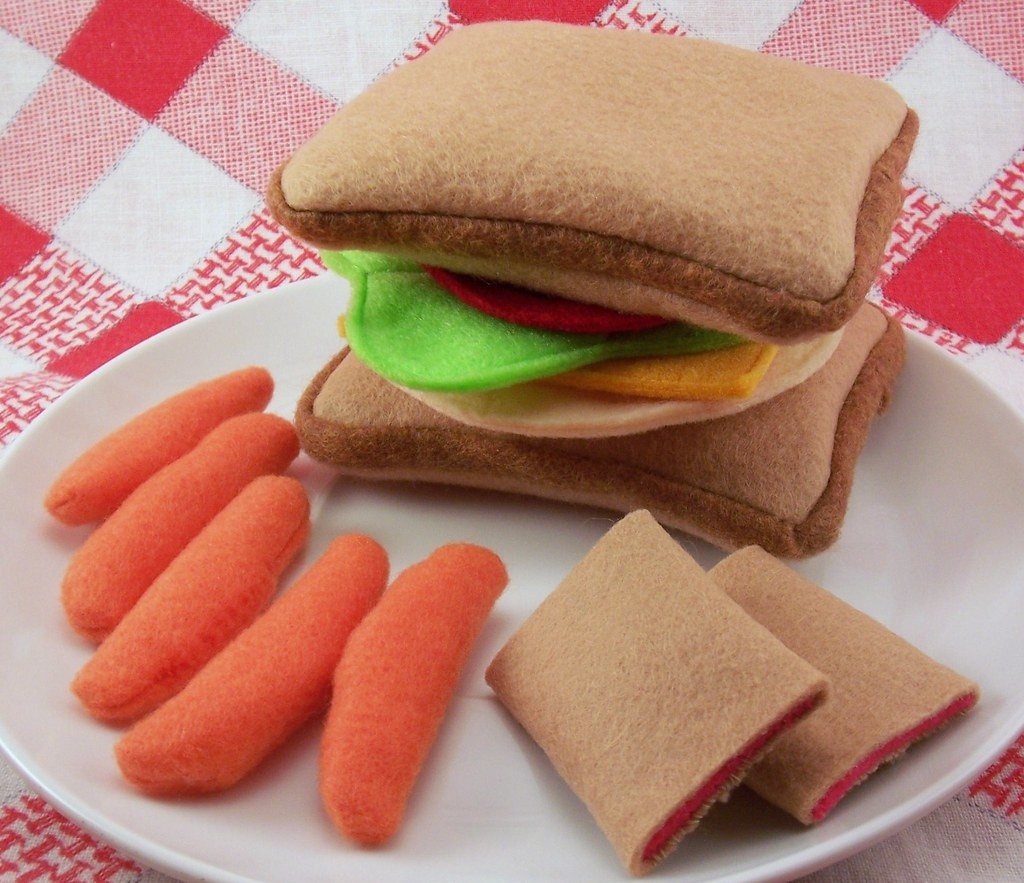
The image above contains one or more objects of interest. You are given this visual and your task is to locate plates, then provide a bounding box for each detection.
[0,277,1024,883]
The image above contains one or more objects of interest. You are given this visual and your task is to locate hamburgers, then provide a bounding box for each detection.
[265,18,916,562]
